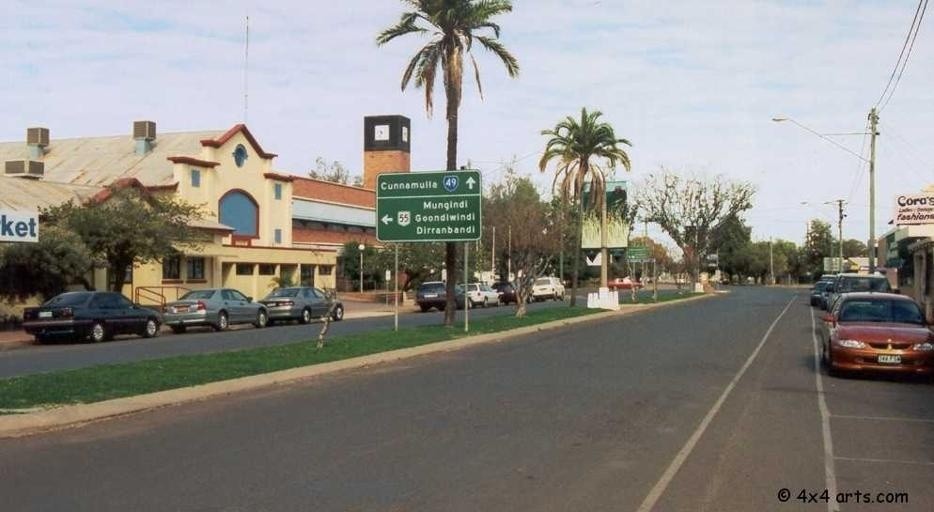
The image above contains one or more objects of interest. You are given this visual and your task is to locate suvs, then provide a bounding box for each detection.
[415,274,565,311]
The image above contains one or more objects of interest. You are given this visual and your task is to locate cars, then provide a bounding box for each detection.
[258,284,343,324]
[162,286,268,332]
[808,272,933,382]
[606,277,643,290]
[21,291,160,344]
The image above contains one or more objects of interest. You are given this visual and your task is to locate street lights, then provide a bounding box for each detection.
[824,199,844,273]
[770,109,878,272]
[356,244,366,293]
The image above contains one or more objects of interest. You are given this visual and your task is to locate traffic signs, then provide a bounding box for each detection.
[374,170,482,243]
